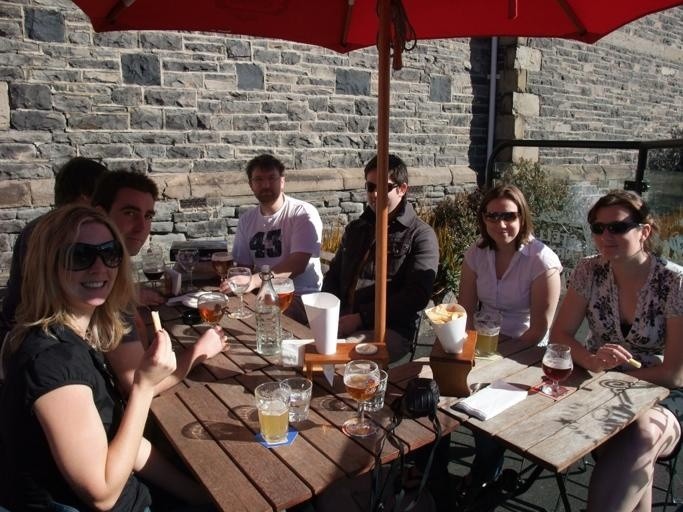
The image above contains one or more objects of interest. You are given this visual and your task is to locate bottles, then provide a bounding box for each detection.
[253,266,286,352]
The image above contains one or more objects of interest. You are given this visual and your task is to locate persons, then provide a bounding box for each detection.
[4,155,106,339]
[549,186,683,512]
[0,207,214,512]
[322,148,443,371]
[447,184,564,494]
[226,149,323,325]
[83,171,229,400]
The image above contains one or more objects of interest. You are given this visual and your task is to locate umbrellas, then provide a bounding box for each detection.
[73,0,681,343]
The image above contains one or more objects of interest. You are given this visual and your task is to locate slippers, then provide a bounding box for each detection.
[394,467,425,491]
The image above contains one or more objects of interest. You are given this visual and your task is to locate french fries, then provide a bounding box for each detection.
[426,304,463,324]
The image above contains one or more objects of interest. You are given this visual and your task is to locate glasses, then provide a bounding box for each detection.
[364,182,399,193]
[59,240,124,271]
[248,175,282,184]
[590,222,637,234]
[483,211,521,222]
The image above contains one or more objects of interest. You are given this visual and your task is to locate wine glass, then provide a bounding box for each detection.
[175,248,202,293]
[343,362,381,435]
[212,250,234,286]
[140,246,167,302]
[226,268,256,319]
[197,293,231,352]
[543,344,575,392]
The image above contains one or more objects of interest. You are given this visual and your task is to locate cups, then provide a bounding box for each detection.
[357,368,389,409]
[473,313,503,357]
[254,381,296,443]
[281,377,315,420]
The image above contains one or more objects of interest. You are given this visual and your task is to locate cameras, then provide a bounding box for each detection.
[404,378,440,414]
